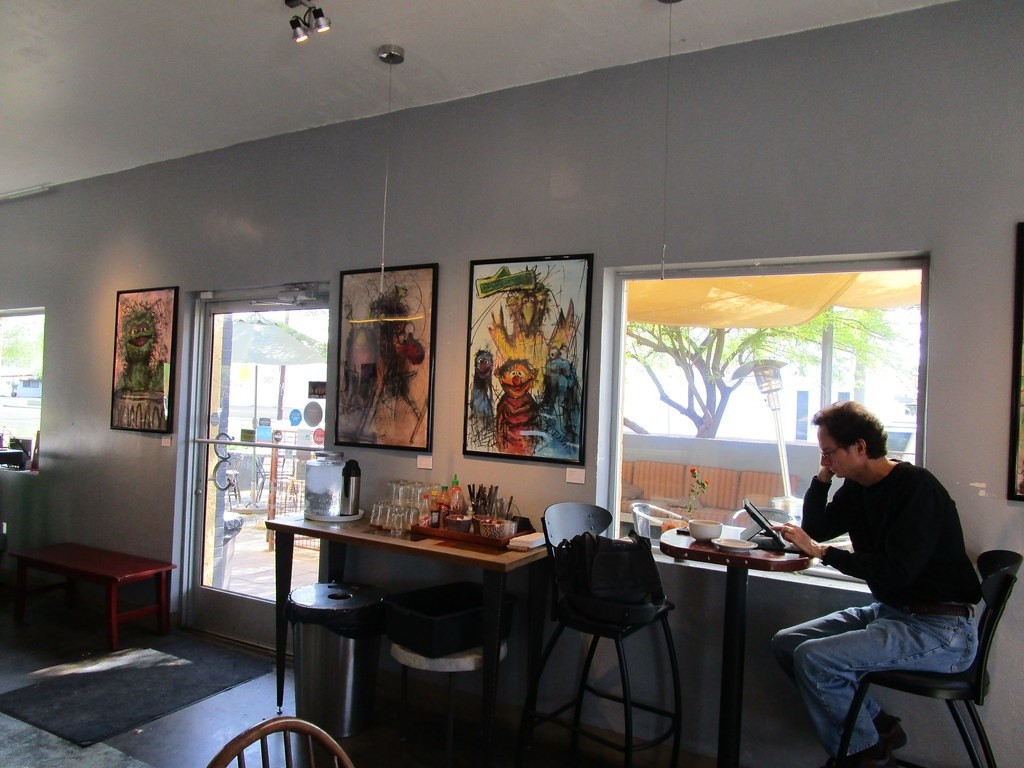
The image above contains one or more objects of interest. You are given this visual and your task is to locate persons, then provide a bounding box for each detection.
[770,401,982,768]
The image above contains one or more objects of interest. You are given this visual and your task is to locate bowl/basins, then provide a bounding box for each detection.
[688,520,723,541]
[446,514,517,538]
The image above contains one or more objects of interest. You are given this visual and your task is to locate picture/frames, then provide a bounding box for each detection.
[333,262,439,453]
[462,252,594,465]
[1006,221,1024,502]
[110,286,179,434]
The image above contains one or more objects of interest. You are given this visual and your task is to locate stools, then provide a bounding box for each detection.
[513,501,683,768]
[390,641,508,768]
[831,550,1024,768]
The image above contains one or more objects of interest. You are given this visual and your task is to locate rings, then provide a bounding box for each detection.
[789,528,792,533]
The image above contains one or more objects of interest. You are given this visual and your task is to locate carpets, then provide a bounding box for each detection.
[0,635,273,747]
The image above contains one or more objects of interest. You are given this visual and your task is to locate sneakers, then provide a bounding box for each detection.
[822,757,894,768]
[863,709,907,751]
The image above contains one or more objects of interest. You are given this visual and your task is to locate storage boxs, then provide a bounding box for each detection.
[382,579,513,659]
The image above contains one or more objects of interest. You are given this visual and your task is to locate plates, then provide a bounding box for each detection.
[711,538,758,553]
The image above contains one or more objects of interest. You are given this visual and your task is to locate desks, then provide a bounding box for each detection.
[265,513,547,768]
[9,543,177,652]
[660,525,814,768]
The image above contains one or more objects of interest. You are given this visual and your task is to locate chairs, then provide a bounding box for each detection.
[630,502,690,546]
[206,717,357,768]
[731,506,795,533]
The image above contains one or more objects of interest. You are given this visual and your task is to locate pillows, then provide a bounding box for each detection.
[622,480,644,498]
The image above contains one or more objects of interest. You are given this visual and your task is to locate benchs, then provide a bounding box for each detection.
[620,460,796,538]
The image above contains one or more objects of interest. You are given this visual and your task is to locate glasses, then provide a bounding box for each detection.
[819,442,847,458]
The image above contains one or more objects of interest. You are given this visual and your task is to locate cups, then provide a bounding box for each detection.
[469,497,529,531]
[370,479,441,536]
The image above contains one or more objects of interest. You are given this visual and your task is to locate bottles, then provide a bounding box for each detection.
[418,474,475,534]
[304,451,346,516]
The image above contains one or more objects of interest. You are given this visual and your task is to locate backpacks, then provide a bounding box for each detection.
[549,531,665,633]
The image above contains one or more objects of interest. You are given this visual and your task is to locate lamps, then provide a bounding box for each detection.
[289,0,332,42]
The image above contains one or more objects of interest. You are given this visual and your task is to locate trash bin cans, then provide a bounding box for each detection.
[283,579,390,738]
[221,511,245,591]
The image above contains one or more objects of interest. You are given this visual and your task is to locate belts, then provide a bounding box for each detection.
[906,604,970,620]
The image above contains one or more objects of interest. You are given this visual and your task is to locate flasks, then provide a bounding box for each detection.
[340,459,362,517]
